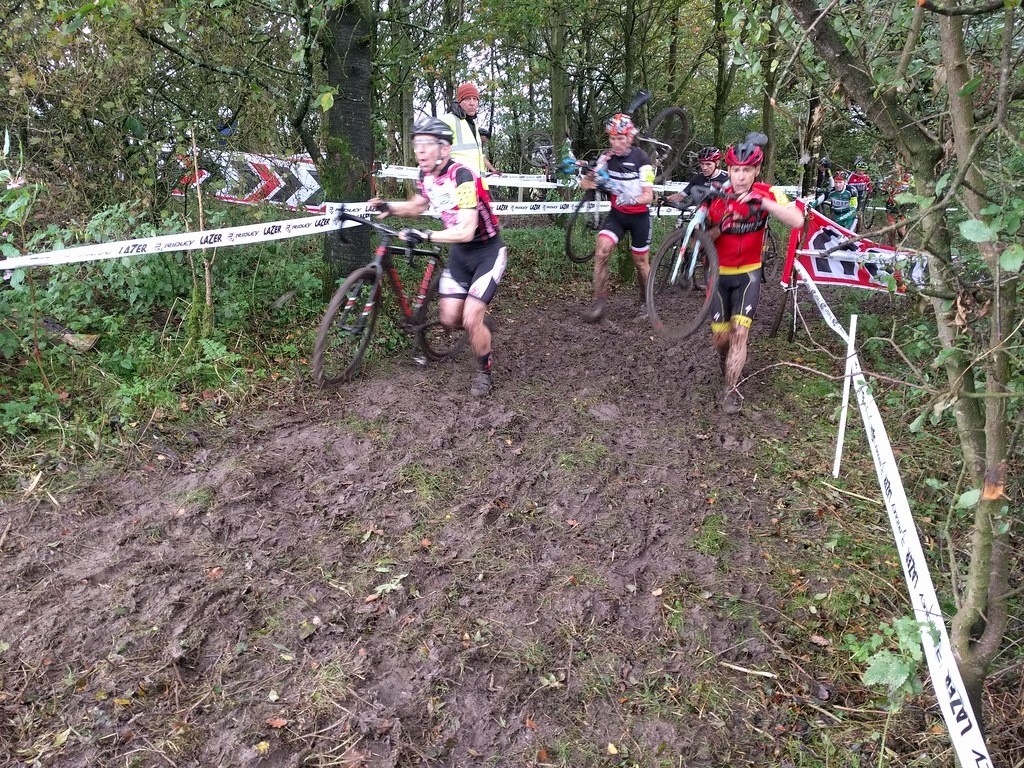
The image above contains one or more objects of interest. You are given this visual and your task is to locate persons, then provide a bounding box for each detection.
[690,140,804,413]
[655,146,730,249]
[432,83,501,201]
[808,144,916,251]
[580,113,661,323]
[364,117,511,399]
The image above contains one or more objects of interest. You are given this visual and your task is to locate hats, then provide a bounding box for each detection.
[456,83,479,103]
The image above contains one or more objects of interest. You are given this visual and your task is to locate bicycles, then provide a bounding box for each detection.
[311,204,470,389]
[760,216,780,285]
[810,177,889,238]
[655,195,709,297]
[647,178,763,345]
[554,92,691,265]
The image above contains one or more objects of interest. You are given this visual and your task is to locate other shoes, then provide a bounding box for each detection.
[721,385,744,414]
[632,304,659,321]
[470,371,492,396]
[482,317,497,344]
[582,292,609,322]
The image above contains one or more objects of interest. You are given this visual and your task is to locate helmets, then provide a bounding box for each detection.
[410,117,455,136]
[725,143,764,166]
[479,128,490,137]
[820,157,831,168]
[833,171,846,180]
[696,147,722,161]
[605,114,637,136]
[855,162,868,171]
